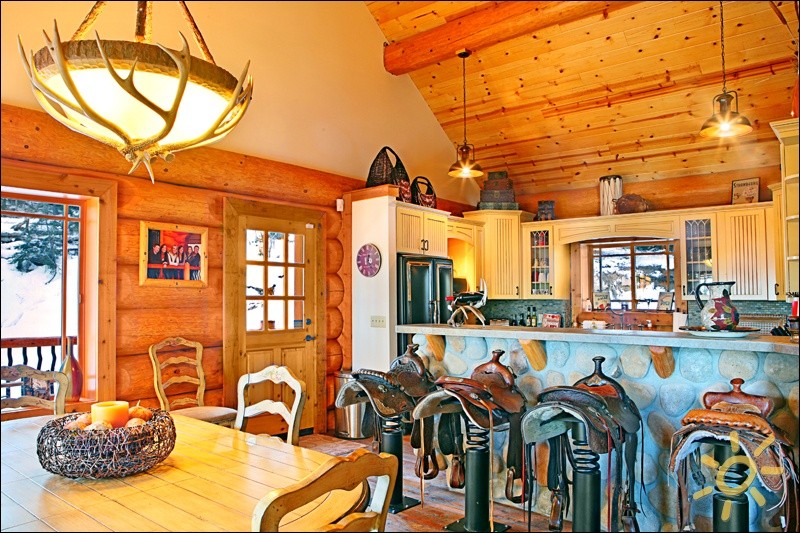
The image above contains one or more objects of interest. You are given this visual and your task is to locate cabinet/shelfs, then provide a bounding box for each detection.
[352,115,800,304]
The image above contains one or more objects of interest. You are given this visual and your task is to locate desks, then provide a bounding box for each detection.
[0,413,370,533]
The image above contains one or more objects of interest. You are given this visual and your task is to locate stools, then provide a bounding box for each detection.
[334,340,795,533]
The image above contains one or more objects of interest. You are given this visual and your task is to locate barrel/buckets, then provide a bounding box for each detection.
[333,370,375,439]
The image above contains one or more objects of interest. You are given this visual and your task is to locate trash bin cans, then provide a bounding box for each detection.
[332,370,375,440]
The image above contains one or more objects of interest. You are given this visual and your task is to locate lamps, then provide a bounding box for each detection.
[698,0,752,138]
[18,0,254,183]
[448,49,484,177]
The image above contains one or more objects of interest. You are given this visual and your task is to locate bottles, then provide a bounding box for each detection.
[531,307,537,327]
[526,307,531,327]
[445,291,484,303]
[58,336,84,403]
[533,231,548,246]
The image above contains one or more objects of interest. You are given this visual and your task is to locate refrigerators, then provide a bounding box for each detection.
[397,253,453,357]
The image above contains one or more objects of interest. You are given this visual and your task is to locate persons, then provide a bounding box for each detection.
[148,244,200,280]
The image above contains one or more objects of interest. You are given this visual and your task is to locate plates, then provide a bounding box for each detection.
[678,325,761,337]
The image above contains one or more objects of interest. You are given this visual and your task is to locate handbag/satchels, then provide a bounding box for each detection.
[409,175,437,209]
[366,146,411,203]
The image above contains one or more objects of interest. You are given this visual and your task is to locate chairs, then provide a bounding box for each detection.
[252,448,398,533]
[148,336,237,429]
[233,365,306,446]
[1,365,68,421]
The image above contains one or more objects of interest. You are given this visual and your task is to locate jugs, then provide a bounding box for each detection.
[695,281,740,332]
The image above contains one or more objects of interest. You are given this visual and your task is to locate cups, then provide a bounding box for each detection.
[792,296,799,316]
[90,400,129,429]
[532,258,550,295]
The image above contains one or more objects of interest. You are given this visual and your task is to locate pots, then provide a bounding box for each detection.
[788,316,799,330]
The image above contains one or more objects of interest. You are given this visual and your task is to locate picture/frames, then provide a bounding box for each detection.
[139,220,209,287]
[731,177,759,204]
